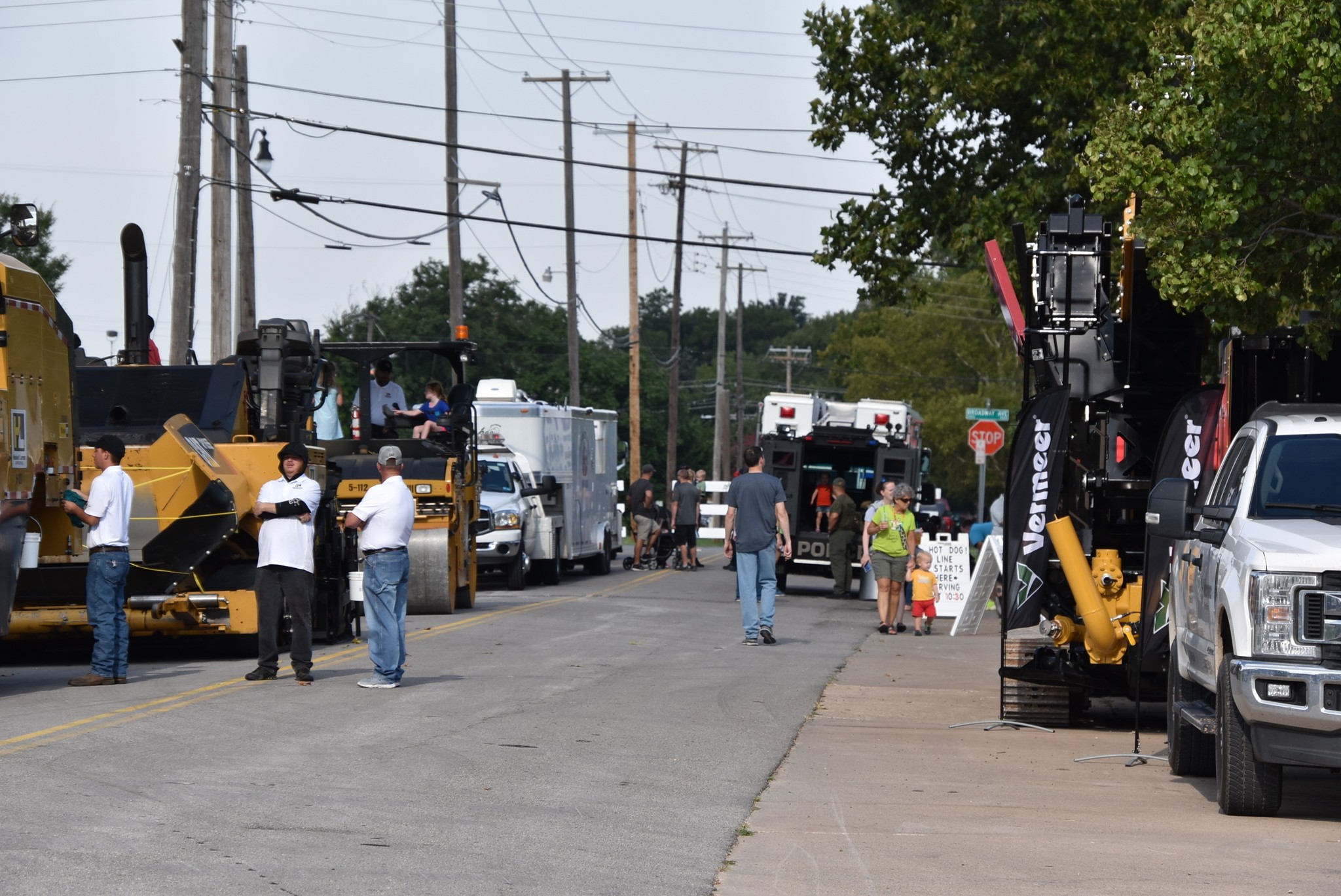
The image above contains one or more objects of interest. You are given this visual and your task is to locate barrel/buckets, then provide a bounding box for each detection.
[19,516,42,568]
[347,561,363,601]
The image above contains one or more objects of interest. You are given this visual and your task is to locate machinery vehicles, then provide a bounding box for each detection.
[0,219,486,662]
[998,188,1334,731]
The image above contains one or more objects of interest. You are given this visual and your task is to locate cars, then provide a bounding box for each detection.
[916,496,960,540]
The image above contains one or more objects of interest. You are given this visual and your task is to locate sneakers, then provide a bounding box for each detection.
[357,674,401,689]
[295,668,314,682]
[245,668,278,681]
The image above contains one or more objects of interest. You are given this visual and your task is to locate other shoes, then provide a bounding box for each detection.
[631,553,704,571]
[723,564,736,571]
[815,528,821,533]
[825,591,853,600]
[897,622,907,632]
[915,621,932,636]
[775,588,785,596]
[984,599,997,611]
[741,624,776,646]
[734,598,741,602]
[68,673,127,687]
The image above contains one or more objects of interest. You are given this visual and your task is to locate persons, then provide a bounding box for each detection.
[245,442,322,682]
[867,482,916,635]
[810,472,834,533]
[344,444,418,689]
[60,434,136,686]
[391,379,452,439]
[724,446,792,645]
[669,462,707,571]
[312,360,344,440]
[905,551,939,636]
[989,491,1005,576]
[851,498,992,610]
[116,314,161,366]
[350,358,407,439]
[827,478,857,599]
[960,519,1004,608]
[860,478,907,633]
[721,463,786,603]
[626,464,662,571]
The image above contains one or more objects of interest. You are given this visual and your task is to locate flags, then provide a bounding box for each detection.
[995,385,1071,632]
[1142,383,1224,674]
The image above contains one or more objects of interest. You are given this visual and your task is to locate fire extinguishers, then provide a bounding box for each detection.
[350,405,361,440]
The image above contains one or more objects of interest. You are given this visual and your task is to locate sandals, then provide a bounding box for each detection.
[879,621,897,635]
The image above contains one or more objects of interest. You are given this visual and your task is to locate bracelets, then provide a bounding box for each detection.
[909,555,916,559]
[827,528,833,531]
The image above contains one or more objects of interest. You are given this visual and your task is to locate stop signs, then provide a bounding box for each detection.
[967,419,1005,456]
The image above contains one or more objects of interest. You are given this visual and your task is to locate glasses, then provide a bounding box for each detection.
[900,498,914,503]
[881,478,886,482]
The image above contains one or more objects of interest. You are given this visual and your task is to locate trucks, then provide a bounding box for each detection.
[749,389,932,600]
[470,377,623,591]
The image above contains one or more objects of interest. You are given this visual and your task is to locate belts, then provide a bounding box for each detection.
[361,546,406,556]
[89,546,129,555]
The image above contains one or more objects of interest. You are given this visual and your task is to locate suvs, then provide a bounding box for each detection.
[1145,395,1341,821]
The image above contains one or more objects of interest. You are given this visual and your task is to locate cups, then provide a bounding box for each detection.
[881,512,889,524]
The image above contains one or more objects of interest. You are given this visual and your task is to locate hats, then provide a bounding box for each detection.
[739,466,749,474]
[377,361,393,373]
[378,444,402,465]
[85,435,126,458]
[642,464,657,474]
[734,471,739,477]
[833,478,847,487]
